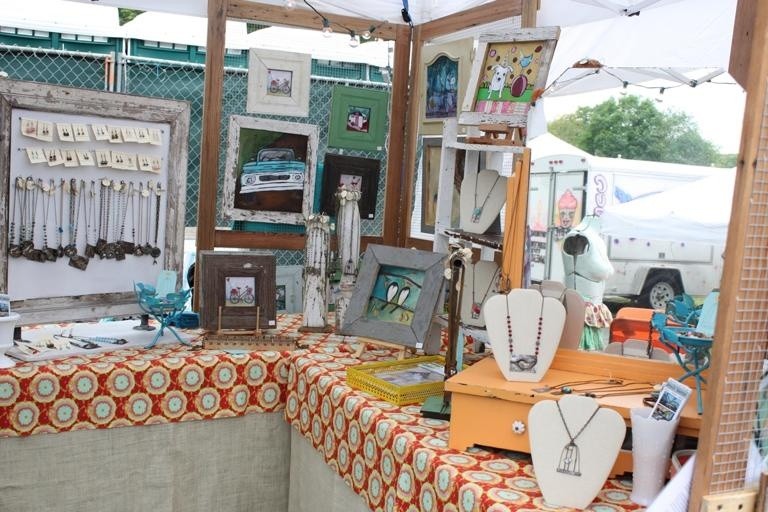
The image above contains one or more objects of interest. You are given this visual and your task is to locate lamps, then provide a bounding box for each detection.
[284,0,388,47]
[620,81,665,104]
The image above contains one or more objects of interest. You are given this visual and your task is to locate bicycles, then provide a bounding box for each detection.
[269,79,291,94]
[230,286,254,304]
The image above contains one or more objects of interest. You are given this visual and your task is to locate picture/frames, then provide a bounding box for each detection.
[277,265,304,312]
[419,36,474,135]
[423,137,501,236]
[343,242,450,356]
[346,353,448,406]
[200,249,276,329]
[219,48,391,225]
[458,26,559,128]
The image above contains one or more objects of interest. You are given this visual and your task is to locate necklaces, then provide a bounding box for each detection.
[471,261,500,320]
[503,294,545,374]
[530,377,656,399]
[300,183,361,329]
[553,400,602,478]
[4,177,164,272]
[471,172,501,226]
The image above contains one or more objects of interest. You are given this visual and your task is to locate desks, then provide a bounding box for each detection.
[0,312,696,512]
[614,307,695,354]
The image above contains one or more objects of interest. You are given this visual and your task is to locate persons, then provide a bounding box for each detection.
[563,212,618,352]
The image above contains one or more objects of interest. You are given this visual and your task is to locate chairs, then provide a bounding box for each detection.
[608,317,664,367]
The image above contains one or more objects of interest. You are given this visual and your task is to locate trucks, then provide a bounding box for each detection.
[597,232,724,313]
